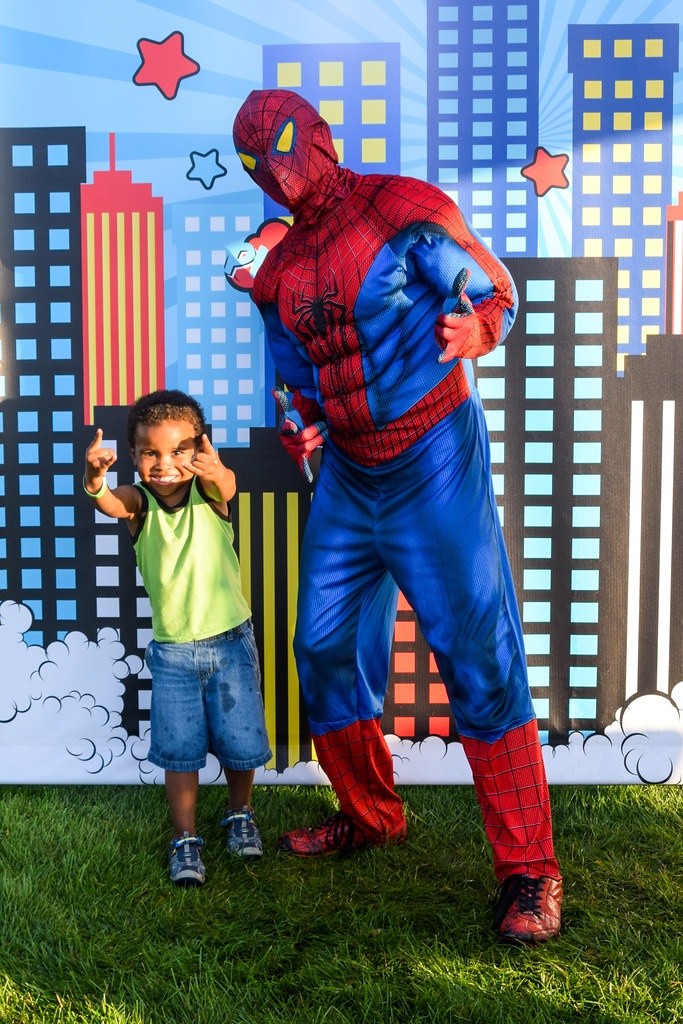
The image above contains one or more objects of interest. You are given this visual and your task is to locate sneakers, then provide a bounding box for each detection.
[491,874,563,945]
[168,831,206,884]
[278,809,408,861]
[220,806,264,857]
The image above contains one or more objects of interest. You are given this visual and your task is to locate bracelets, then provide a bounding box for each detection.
[82,477,106,499]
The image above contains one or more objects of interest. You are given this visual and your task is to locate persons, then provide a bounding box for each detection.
[85,389,274,884]
[231,89,563,943]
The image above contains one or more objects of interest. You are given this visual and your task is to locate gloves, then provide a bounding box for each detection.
[434,267,475,364]
[271,385,329,483]
